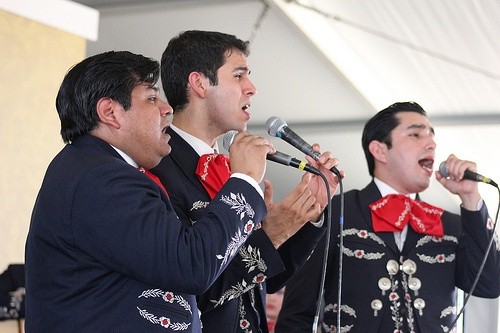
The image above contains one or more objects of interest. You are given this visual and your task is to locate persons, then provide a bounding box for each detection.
[149,30,344,332]
[275,102,499,333]
[25,52,277,333]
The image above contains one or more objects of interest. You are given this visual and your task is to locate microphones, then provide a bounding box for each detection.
[439,161,498,188]
[265,116,340,176]
[266,151,321,175]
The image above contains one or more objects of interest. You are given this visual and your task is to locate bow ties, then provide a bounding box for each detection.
[195,154,231,201]
[137,166,170,201]
[369,193,444,237]
